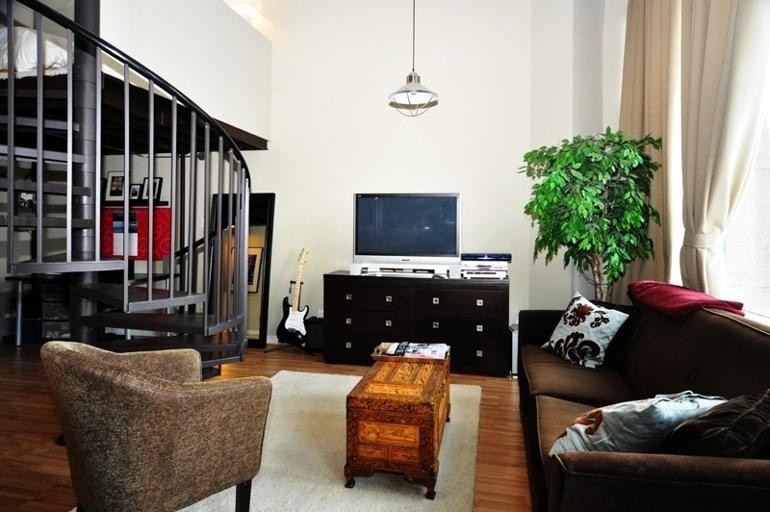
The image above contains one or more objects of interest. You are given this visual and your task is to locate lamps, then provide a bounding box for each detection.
[390,0,435,118]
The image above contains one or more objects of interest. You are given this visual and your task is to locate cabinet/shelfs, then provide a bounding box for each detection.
[321,269,520,378]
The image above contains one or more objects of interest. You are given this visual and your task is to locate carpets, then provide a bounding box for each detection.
[172,365,487,506]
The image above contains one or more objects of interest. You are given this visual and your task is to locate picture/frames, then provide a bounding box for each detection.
[226,245,264,294]
[103,169,164,202]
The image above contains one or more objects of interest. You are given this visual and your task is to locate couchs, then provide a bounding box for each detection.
[516,292,770,512]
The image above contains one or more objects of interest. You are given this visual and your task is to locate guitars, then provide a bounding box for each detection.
[276,248,312,353]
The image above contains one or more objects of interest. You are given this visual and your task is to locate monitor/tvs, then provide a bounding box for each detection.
[350,192,462,281]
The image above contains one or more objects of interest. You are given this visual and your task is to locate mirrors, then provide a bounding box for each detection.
[204,191,277,348]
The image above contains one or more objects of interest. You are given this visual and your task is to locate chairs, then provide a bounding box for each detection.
[41,339,274,507]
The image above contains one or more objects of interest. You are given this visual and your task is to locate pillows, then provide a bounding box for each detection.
[538,383,769,461]
[538,291,629,373]
